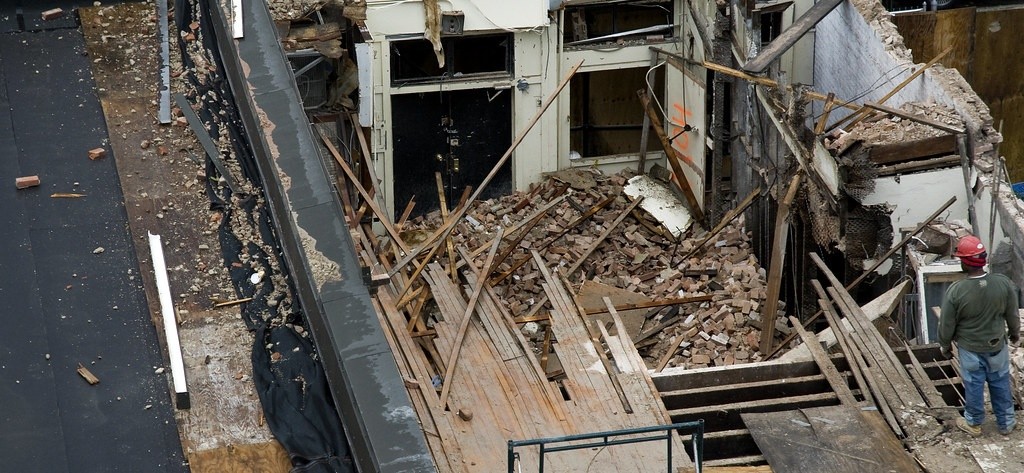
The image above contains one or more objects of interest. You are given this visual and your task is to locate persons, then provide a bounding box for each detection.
[935,235,1022,436]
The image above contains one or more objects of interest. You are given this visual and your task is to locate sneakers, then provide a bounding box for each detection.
[956,417,982,436]
[1000,420,1018,434]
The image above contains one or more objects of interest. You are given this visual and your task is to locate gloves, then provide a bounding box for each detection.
[939,345,954,359]
[1007,330,1020,344]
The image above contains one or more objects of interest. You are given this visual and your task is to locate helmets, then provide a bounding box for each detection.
[951,235,985,257]
